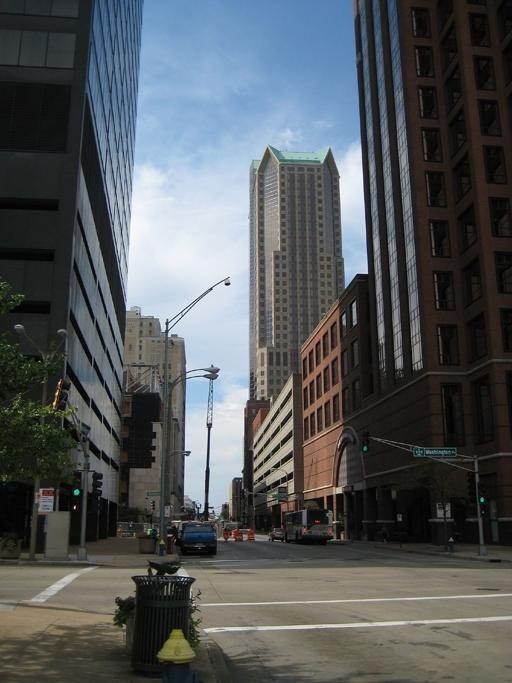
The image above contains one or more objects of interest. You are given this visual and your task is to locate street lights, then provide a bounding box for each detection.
[13,324,68,561]
[162,448,191,519]
[266,465,288,513]
[162,363,223,540]
[152,270,235,557]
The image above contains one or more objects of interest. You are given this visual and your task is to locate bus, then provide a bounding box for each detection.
[282,506,333,545]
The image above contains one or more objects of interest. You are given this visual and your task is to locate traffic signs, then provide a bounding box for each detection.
[411,446,457,457]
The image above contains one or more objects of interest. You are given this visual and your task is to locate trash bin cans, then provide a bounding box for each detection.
[165,533,176,553]
[131,575,196,673]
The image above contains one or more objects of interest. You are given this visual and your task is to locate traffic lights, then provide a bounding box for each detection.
[239,488,245,500]
[150,499,155,510]
[93,471,103,499]
[52,377,72,411]
[477,495,487,518]
[72,470,82,499]
[361,430,368,453]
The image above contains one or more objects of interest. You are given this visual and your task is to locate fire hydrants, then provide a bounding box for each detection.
[158,538,165,556]
[449,535,456,551]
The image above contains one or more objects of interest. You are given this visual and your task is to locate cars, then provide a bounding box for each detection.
[267,527,286,541]
[168,517,217,555]
[219,518,243,534]
